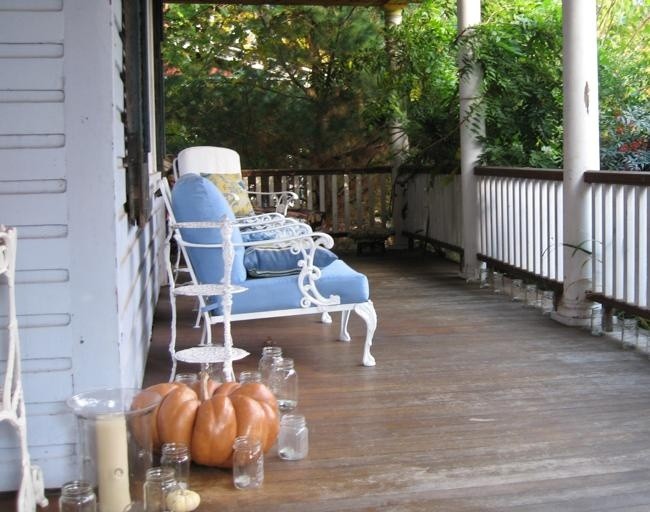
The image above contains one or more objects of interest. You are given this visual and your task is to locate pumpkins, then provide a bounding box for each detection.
[127,372,282,471]
[164,485,202,512]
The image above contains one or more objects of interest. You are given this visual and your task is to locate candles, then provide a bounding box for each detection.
[94,412,132,512]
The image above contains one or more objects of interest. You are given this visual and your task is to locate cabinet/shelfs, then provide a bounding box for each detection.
[0,229,54,512]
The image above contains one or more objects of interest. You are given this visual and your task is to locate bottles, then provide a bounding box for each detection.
[239,370,263,386]
[160,442,191,491]
[269,358,298,412]
[175,373,197,387]
[276,414,310,461]
[141,466,181,512]
[257,346,283,385]
[56,479,97,512]
[232,435,265,491]
